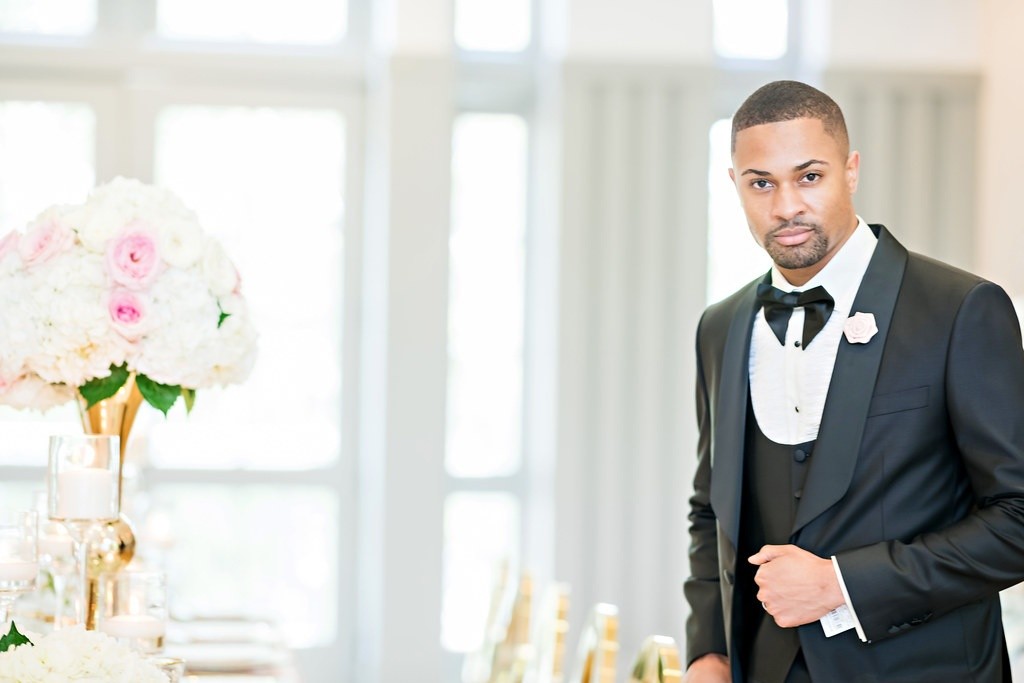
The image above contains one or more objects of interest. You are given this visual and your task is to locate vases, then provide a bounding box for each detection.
[43,375,145,620]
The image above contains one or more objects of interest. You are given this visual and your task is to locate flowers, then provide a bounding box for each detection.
[1,172,279,418]
[0,619,172,683]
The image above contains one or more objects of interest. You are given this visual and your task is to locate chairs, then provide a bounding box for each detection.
[458,564,678,683]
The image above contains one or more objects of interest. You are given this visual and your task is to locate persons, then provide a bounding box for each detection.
[681,80,1024,683]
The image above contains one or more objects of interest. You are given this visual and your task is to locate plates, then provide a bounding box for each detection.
[159,599,284,671]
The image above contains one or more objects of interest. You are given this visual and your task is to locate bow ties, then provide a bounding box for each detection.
[757,283,835,350]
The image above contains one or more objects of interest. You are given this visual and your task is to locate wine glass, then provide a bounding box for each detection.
[0,431,150,644]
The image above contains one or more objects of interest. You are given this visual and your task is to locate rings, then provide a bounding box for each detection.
[762,603,767,610]
